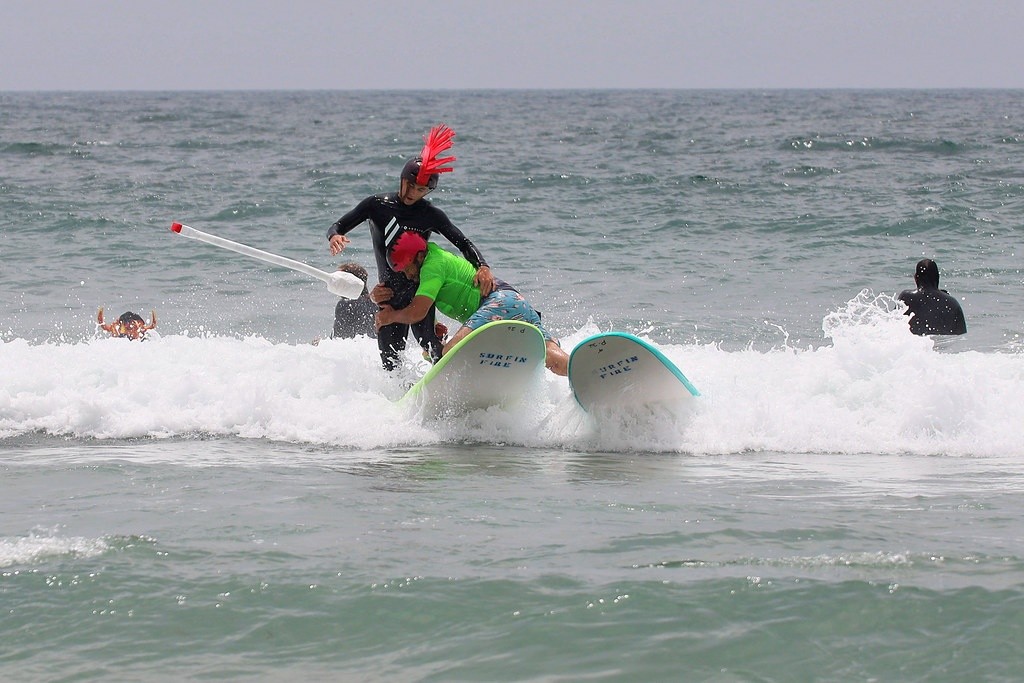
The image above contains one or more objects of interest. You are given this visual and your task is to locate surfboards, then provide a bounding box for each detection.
[392,317,547,428]
[567,330,701,429]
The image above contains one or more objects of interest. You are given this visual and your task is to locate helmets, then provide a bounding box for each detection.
[401,124,456,190]
[386,230,427,273]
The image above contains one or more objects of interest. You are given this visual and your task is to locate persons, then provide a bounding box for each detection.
[335,264,379,339]
[892,259,967,335]
[326,159,496,378]
[368,226,569,376]
